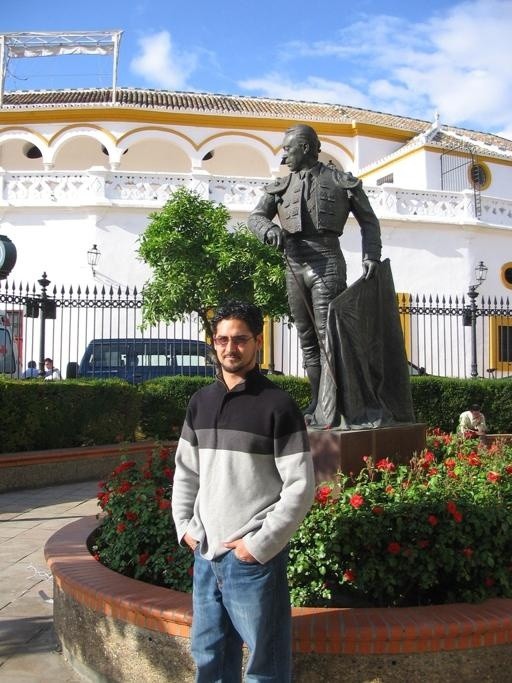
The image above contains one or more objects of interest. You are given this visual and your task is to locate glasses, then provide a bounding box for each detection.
[212,336,252,348]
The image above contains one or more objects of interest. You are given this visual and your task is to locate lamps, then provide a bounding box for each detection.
[471,261,488,291]
[87,244,101,276]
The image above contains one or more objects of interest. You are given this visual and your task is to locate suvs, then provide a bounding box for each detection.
[66,338,284,386]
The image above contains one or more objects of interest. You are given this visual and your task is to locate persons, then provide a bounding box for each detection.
[20,360,40,378]
[457,402,487,436]
[43,358,61,380]
[246,123,382,416]
[171,305,317,683]
